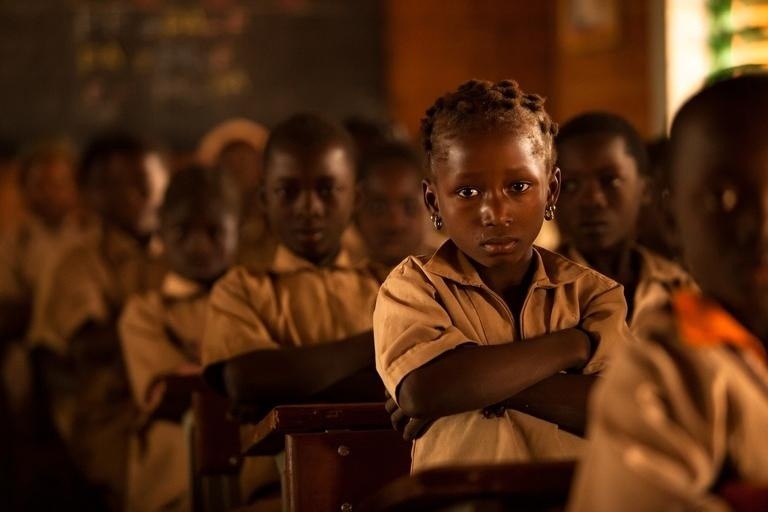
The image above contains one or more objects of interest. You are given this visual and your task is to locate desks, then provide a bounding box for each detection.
[238,402,394,458]
[360,455,576,511]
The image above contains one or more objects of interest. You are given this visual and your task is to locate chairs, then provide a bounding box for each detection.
[283,428,412,512]
[186,388,245,510]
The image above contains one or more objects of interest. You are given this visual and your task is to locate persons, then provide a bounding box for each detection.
[371,76,633,469]
[560,64,767,511]
